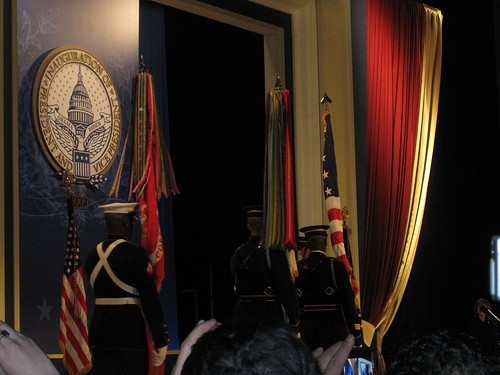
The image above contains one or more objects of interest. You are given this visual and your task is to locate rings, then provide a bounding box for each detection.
[0,330,9,339]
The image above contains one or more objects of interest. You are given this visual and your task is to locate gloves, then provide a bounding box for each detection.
[153,345,168,367]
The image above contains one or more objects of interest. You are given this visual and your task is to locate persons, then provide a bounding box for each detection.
[85,202,169,375]
[229,208,300,337]
[172,319,323,375]
[0,320,60,375]
[313,330,500,375]
[294,225,361,351]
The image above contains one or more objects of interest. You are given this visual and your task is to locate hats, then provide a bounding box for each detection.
[300,224,330,238]
[247,209,263,217]
[298,236,307,243]
[98,201,139,224]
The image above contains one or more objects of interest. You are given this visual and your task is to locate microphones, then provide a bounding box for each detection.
[480,304,500,325]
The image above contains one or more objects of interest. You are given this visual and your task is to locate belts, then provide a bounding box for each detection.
[238,294,276,302]
[303,304,338,312]
[95,297,137,305]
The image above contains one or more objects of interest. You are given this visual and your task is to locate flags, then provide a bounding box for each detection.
[323,112,359,308]
[57,191,93,375]
